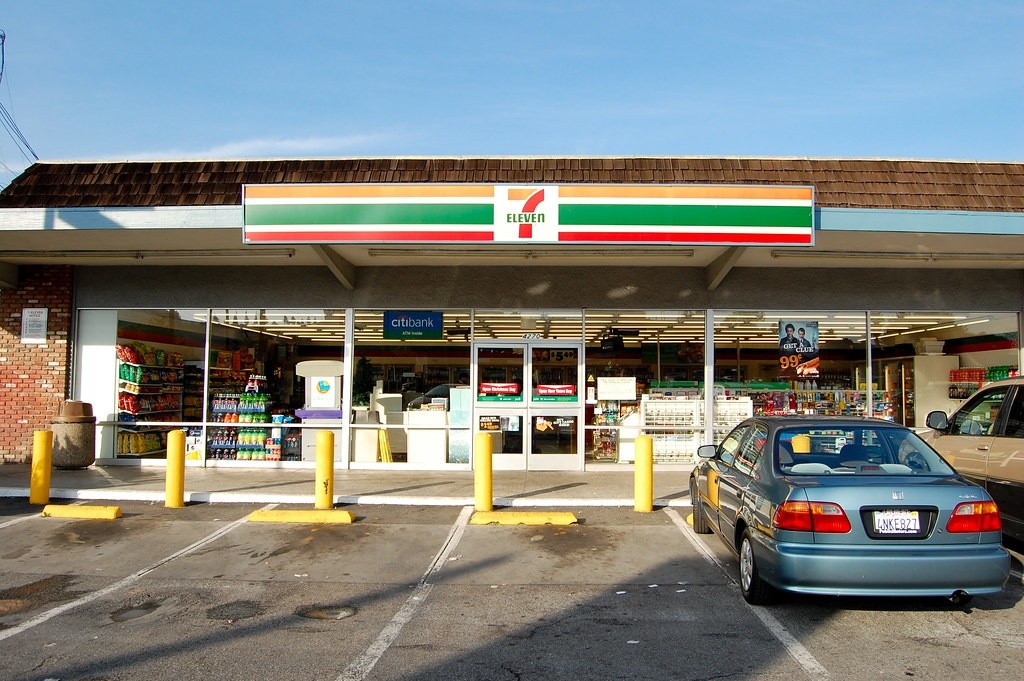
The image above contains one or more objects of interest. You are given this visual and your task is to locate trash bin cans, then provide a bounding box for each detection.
[50,398,96,468]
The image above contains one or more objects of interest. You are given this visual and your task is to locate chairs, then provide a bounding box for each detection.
[840,444,868,464]
[779,442,795,463]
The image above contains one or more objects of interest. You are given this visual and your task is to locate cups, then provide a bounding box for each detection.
[798,382,803,390]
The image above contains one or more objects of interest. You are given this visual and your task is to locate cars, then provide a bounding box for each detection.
[689,415,1012,605]
[406,384,468,411]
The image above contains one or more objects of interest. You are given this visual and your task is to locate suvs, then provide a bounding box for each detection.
[898,376,1024,543]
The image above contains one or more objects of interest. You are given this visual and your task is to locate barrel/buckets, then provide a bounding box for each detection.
[835,437,846,448]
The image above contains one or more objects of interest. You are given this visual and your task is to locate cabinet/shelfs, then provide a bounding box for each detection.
[115,358,1018,471]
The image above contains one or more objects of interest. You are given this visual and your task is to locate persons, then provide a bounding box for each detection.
[781,324,811,347]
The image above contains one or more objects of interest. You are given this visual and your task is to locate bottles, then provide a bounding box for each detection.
[949,383,981,399]
[237,428,267,445]
[794,381,798,390]
[237,448,281,461]
[240,393,271,408]
[248,379,268,389]
[949,365,1020,382]
[805,380,811,391]
[812,380,817,390]
[267,438,273,445]
[224,413,270,423]
